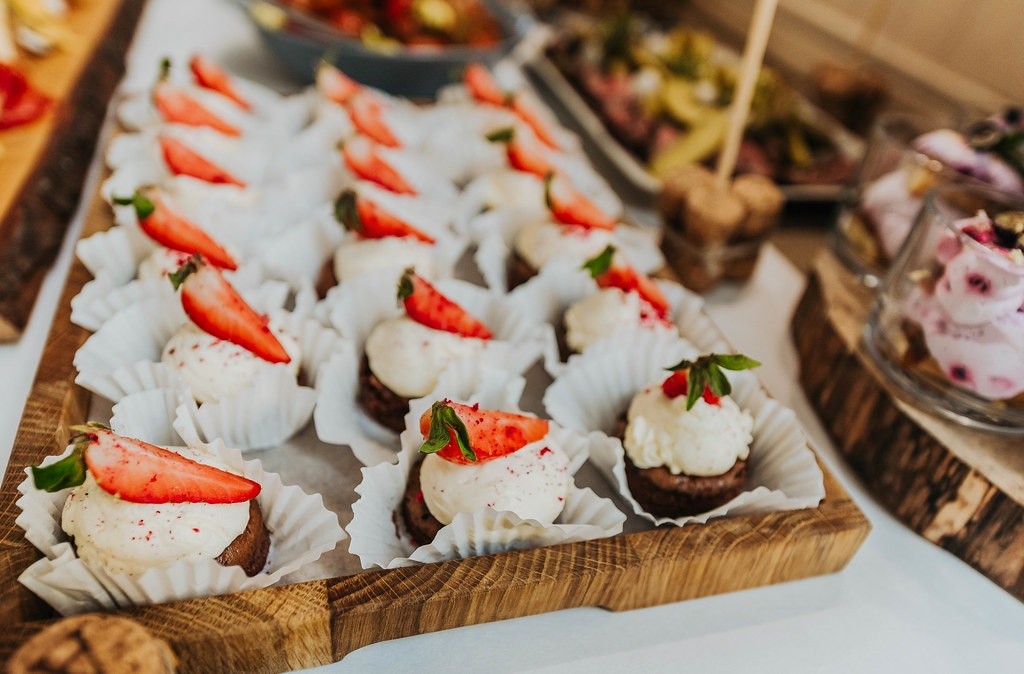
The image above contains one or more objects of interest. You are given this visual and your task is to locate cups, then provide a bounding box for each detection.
[826,107,1024,291]
[856,179,1024,435]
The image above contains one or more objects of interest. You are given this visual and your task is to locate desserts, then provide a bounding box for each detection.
[12,51,825,615]
[836,100,1024,433]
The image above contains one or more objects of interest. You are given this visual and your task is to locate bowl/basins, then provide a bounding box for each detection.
[237,0,534,104]
